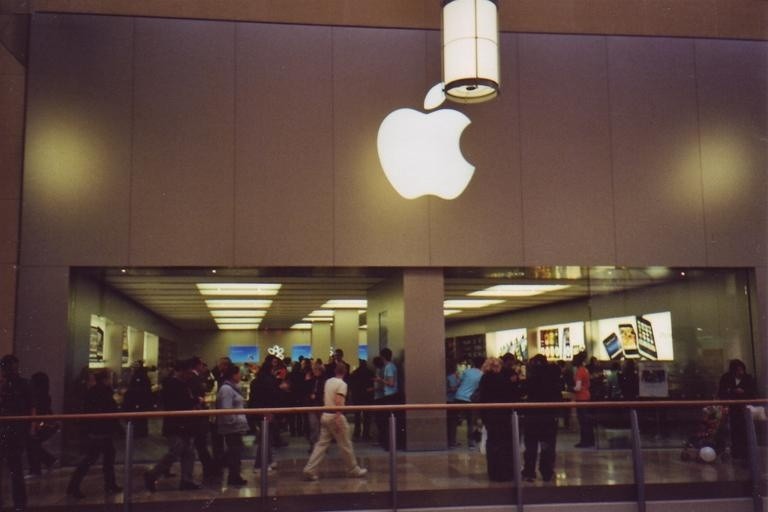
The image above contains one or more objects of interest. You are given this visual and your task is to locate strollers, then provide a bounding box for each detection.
[679,406,731,465]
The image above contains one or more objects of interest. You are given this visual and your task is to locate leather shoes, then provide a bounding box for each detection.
[180,478,203,491]
[227,476,248,486]
[143,471,157,493]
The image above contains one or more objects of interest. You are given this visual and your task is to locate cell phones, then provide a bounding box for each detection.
[617,323,641,360]
[635,315,658,360]
[90,326,99,361]
[97,326,104,361]
[603,332,623,360]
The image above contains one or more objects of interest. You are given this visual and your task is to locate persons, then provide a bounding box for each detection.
[446,351,639,448]
[479,353,563,481]
[2,355,53,509]
[126,348,400,489]
[678,358,758,459]
[66,370,124,496]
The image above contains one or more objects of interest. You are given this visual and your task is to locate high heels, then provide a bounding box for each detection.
[66,481,86,498]
[104,482,122,493]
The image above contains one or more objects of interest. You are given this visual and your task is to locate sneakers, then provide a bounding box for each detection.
[346,465,367,477]
[521,468,537,481]
[303,466,319,480]
[543,471,557,480]
